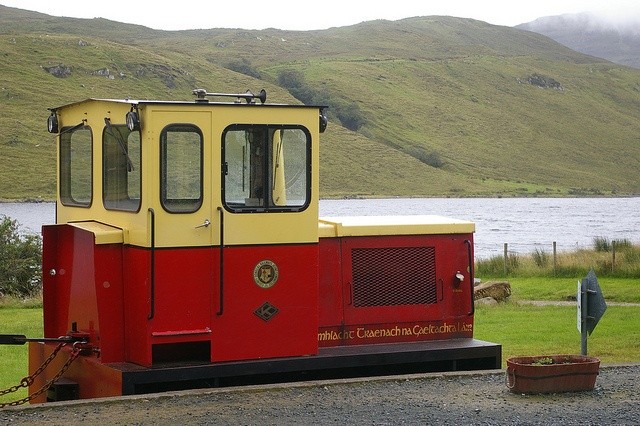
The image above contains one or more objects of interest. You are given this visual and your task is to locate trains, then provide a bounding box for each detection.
[26,87,505,408]
